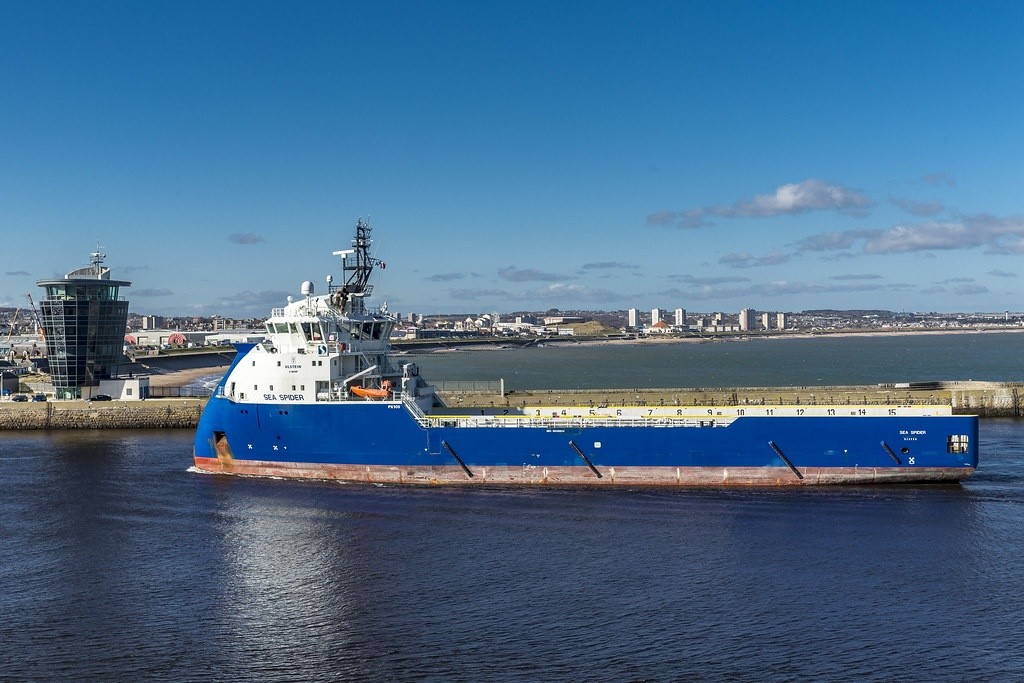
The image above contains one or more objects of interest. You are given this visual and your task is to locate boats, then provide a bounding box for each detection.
[194,216,981,487]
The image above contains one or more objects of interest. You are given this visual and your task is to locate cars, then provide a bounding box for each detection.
[12,395,28,402]
[0,388,12,396]
[85,395,112,401]
[31,395,47,403]
[133,337,233,351]
